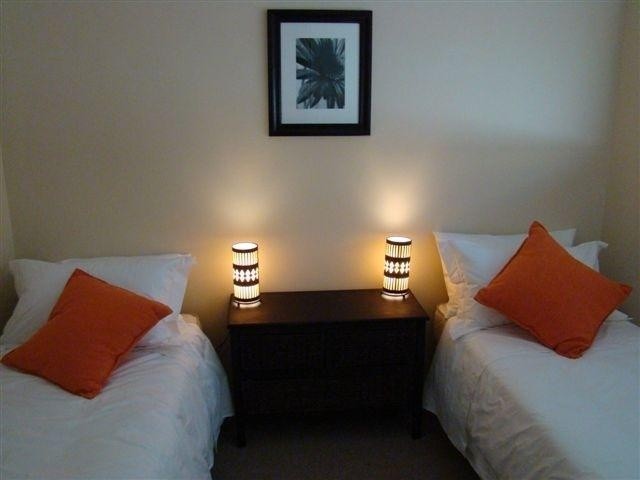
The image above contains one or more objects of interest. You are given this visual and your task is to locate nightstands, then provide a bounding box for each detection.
[225,287,432,441]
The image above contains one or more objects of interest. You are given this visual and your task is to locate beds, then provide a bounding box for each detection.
[0,314,235,479]
[423,300,640,480]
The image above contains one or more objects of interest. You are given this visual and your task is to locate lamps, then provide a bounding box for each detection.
[231,241,264,308]
[380,236,412,301]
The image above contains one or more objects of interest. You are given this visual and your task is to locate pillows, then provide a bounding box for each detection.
[1,268,174,401]
[430,227,578,320]
[3,253,196,352]
[472,220,634,361]
[448,238,629,342]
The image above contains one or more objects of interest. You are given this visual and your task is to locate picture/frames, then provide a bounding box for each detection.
[267,8,373,137]
